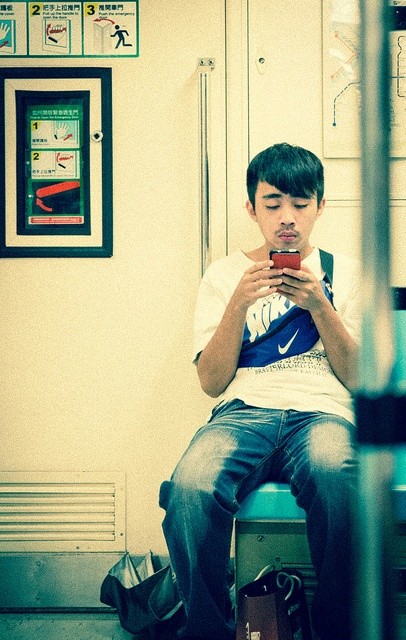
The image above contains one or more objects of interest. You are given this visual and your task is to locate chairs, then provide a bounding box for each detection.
[233,308,405,640]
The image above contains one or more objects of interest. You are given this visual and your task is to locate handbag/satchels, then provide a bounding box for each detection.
[237,568,304,639]
[238,280,331,366]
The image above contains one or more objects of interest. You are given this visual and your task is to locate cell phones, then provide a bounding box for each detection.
[269,249,301,292]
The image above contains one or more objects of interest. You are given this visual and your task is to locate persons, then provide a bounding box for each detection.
[158,142,395,640]
